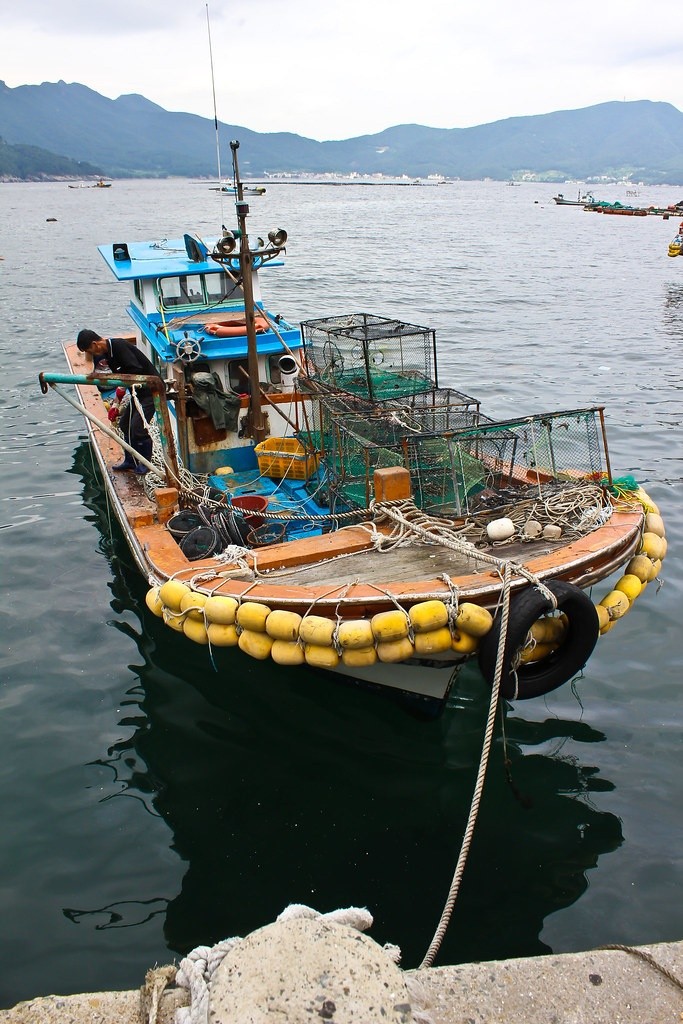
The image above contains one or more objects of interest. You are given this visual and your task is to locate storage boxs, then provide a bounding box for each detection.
[299,311,613,522]
[253,437,321,481]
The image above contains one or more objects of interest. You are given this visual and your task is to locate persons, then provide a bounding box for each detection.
[77,329,165,474]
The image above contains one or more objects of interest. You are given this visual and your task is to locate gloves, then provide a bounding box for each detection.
[116,386,126,401]
[108,403,119,422]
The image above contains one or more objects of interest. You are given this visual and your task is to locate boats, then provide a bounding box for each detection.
[38,2,667,706]
[209,180,266,196]
[92,178,112,188]
[548,187,683,260]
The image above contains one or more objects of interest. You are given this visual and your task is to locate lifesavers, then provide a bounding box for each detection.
[197,316,271,337]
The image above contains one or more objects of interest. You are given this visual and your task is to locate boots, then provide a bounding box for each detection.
[112,436,138,470]
[133,438,153,473]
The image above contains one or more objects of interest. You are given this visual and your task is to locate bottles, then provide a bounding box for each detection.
[96,359,116,389]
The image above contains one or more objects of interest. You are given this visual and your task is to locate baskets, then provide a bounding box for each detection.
[254,437,320,480]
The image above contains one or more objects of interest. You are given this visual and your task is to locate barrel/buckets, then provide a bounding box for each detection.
[167,495,285,561]
